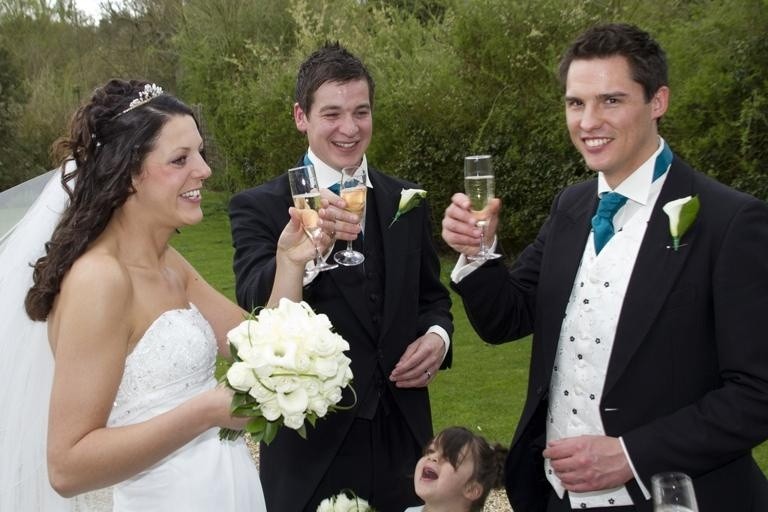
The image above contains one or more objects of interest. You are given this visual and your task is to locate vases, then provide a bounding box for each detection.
[210,293,356,448]
[662,192,702,251]
[388,185,430,228]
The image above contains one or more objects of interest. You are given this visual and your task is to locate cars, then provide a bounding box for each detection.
[650,472,697,512]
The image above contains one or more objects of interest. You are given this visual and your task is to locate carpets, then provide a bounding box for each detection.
[588,137,673,257]
[325,179,365,249]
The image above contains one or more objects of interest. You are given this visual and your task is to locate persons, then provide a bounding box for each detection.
[443,22,768,512]
[394,422,511,512]
[223,36,458,512]
[0,78,270,512]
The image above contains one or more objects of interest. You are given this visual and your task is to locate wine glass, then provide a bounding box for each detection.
[424,369,433,380]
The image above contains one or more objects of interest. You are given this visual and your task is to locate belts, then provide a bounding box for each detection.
[464,155,504,262]
[333,167,367,267]
[288,165,339,273]
